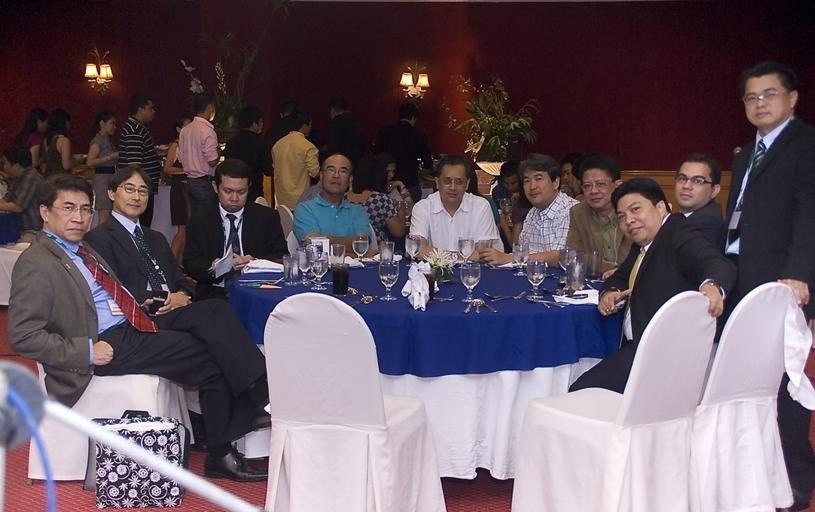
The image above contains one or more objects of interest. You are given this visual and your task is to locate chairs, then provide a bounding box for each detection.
[511,291,719,512]
[694,282,798,512]
[23,358,189,484]
[262,293,447,512]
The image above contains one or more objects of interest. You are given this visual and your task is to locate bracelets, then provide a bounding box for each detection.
[398,184,410,199]
[706,282,718,287]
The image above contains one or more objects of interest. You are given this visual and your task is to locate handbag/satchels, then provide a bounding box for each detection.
[82,418,191,492]
[95,416,181,511]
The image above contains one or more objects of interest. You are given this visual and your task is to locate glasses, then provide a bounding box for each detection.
[48,204,96,217]
[116,185,148,196]
[581,181,614,190]
[675,175,713,187]
[322,168,350,177]
[743,90,787,103]
[438,178,467,188]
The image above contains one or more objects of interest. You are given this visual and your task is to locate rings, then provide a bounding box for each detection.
[389,184,393,188]
[605,308,615,313]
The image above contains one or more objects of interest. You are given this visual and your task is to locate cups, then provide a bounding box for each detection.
[380,240,394,262]
[331,243,346,265]
[332,263,350,298]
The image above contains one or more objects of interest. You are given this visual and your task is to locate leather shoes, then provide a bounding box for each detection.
[204,450,269,482]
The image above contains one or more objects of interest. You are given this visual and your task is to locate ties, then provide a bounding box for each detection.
[133,226,168,291]
[725,138,766,256]
[224,214,241,298]
[73,246,160,333]
[618,247,646,350]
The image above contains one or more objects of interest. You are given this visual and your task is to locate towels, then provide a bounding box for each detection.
[496,261,527,269]
[327,256,365,269]
[403,261,438,315]
[552,289,626,309]
[361,255,401,265]
[241,259,285,272]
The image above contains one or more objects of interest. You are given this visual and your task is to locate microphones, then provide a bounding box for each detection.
[734,145,742,154]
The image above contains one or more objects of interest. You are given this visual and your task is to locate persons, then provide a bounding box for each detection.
[560,154,586,202]
[6,175,269,484]
[266,100,321,161]
[298,153,380,260]
[722,60,815,511]
[298,133,404,249]
[496,181,531,251]
[568,154,634,277]
[311,99,370,192]
[478,153,580,266]
[405,157,505,266]
[381,158,417,216]
[88,111,120,228]
[372,99,433,204]
[669,155,727,249]
[272,111,320,217]
[490,159,528,209]
[222,107,274,208]
[0,145,46,248]
[568,178,739,393]
[17,107,50,170]
[185,157,286,303]
[39,109,80,189]
[176,92,220,221]
[162,115,192,265]
[117,94,161,230]
[86,166,228,451]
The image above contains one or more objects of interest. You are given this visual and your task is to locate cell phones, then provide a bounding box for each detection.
[148,288,169,315]
[612,295,629,307]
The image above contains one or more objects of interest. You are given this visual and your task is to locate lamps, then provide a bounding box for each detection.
[83,49,113,90]
[395,62,431,100]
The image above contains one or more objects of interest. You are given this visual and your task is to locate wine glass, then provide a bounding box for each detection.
[479,240,493,268]
[378,261,399,302]
[458,263,481,303]
[352,236,369,264]
[558,247,577,288]
[588,251,601,286]
[458,238,475,267]
[405,236,421,267]
[310,253,329,291]
[526,260,546,298]
[499,198,510,214]
[296,248,313,284]
[513,242,528,278]
[282,255,299,285]
[567,262,585,297]
[502,205,514,227]
